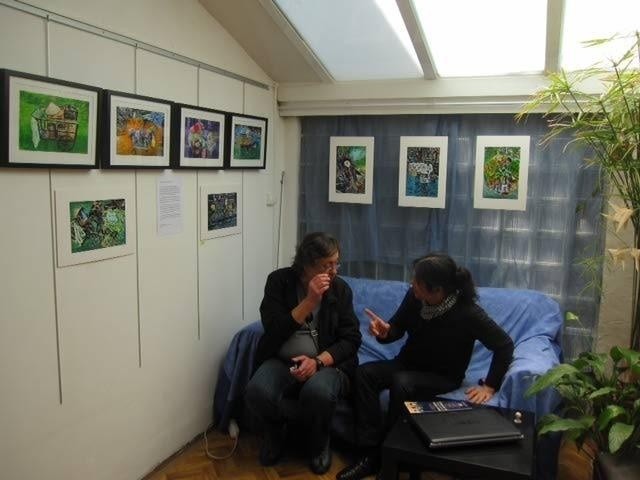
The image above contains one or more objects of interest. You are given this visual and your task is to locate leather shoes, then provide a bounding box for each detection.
[335,455,378,479]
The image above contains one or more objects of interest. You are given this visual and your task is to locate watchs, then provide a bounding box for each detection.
[312,357,325,371]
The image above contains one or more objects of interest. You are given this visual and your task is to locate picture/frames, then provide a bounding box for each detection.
[397,137,448,209]
[329,136,374,205]
[173,103,227,170]
[0,68,100,169]
[228,112,268,170]
[473,136,530,213]
[102,88,172,169]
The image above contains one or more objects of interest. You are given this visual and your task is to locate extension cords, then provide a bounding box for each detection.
[228,418,240,440]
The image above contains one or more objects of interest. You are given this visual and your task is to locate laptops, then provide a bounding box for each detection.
[410,407,525,451]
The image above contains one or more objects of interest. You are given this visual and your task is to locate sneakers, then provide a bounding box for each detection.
[310,436,331,474]
[259,433,285,465]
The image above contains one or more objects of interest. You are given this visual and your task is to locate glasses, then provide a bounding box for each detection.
[315,257,343,271]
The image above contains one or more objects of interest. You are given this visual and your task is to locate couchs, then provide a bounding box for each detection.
[214,276,568,480]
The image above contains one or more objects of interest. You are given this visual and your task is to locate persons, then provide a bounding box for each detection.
[334,254,515,479]
[243,233,362,475]
